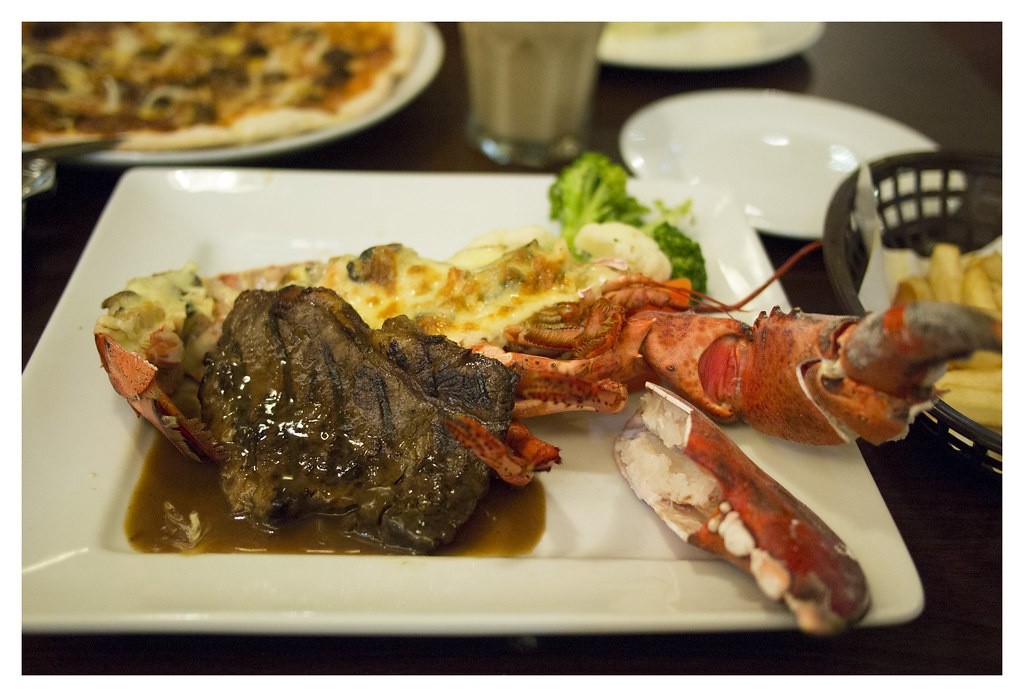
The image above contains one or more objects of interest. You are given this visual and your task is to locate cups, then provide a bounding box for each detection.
[459,21,606,170]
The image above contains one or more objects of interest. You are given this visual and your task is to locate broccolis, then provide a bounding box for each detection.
[649,221,706,296]
[547,152,648,256]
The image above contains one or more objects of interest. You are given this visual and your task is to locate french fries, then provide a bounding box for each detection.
[893,245,1003,435]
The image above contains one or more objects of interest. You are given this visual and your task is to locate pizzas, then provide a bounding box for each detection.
[22,22,418,151]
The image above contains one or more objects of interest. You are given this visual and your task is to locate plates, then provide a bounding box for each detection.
[617,88,968,245]
[21,21,447,163]
[590,22,828,72]
[22,165,923,638]
